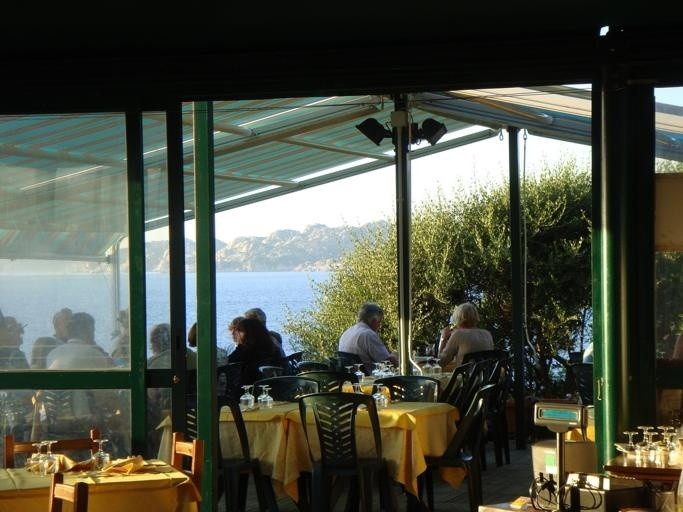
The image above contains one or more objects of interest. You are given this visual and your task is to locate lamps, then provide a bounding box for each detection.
[411,116,447,147]
[355,117,397,147]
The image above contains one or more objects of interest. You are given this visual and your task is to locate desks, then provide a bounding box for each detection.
[1,458,187,512]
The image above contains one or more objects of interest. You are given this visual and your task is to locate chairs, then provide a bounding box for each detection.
[0,383,161,468]
[163,350,510,512]
[46,472,87,512]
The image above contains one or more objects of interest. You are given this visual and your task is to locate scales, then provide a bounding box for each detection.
[534,404,654,512]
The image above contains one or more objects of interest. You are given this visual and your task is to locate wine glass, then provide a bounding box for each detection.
[622,426,683,467]
[91,439,109,474]
[29,443,47,476]
[351,382,386,409]
[41,440,57,473]
[341,346,441,383]
[240,383,272,411]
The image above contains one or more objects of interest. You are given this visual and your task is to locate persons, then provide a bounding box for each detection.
[226,307,294,402]
[338,301,399,369]
[434,301,493,368]
[0,308,232,462]
